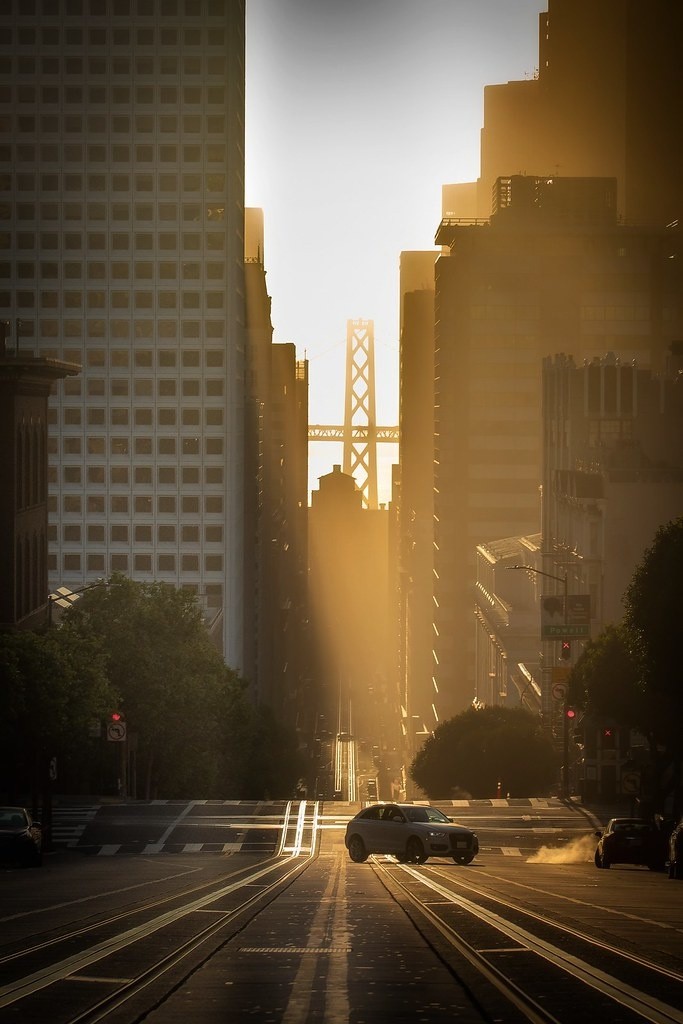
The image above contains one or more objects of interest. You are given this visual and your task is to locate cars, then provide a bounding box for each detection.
[344,803,478,866]
[0,806,42,869]
[592,817,666,872]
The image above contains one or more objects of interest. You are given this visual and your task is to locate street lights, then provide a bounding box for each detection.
[504,565,570,797]
[47,583,127,629]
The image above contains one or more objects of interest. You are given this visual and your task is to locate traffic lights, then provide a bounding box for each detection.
[602,725,613,743]
[564,709,578,723]
[106,688,128,723]
[560,639,571,663]
